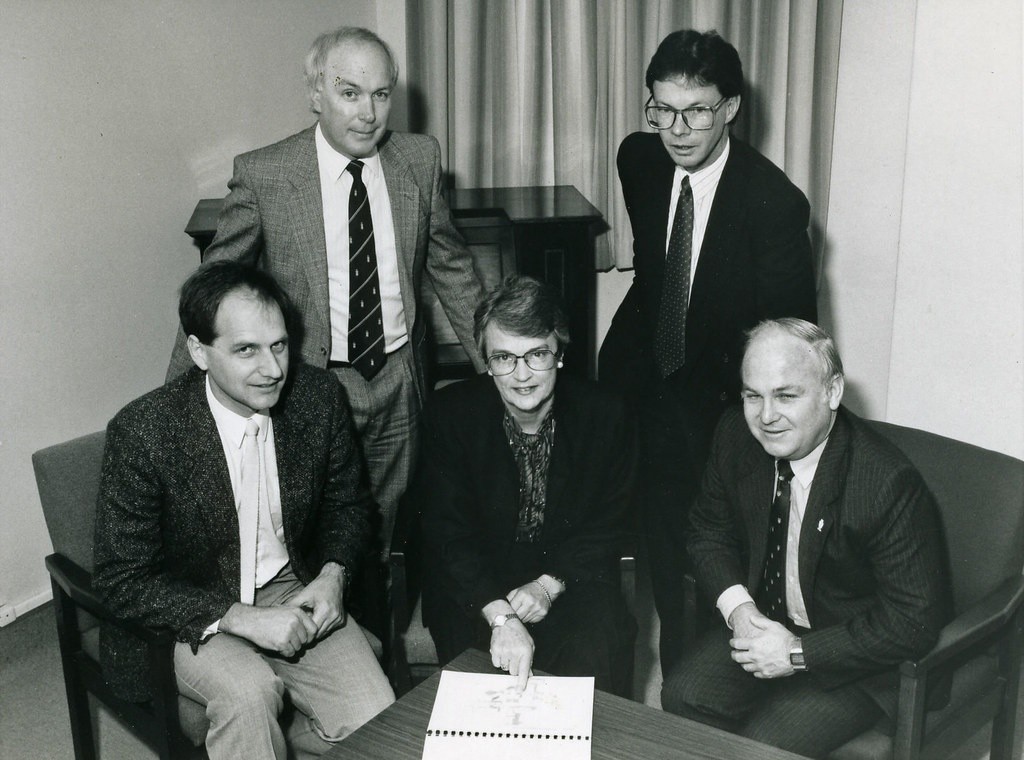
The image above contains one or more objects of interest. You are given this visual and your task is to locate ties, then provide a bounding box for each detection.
[501,408,554,547]
[238,420,262,606]
[345,160,388,382]
[654,175,695,379]
[757,458,793,630]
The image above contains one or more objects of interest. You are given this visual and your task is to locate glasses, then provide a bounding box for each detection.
[645,94,726,131]
[486,350,560,375]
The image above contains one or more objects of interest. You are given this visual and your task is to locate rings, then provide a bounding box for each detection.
[325,631,330,633]
[501,665,508,669]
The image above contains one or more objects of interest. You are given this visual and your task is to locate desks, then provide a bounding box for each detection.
[315,646,812,760]
[186,185,612,388]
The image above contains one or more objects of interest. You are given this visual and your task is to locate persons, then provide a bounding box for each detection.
[95,258,398,759]
[598,29,817,691]
[411,275,638,705]
[167,23,487,655]
[660,318,948,760]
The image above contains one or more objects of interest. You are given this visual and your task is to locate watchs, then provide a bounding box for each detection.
[790,636,808,672]
[490,612,518,631]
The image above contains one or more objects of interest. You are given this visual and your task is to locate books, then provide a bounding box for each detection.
[422,667,596,760]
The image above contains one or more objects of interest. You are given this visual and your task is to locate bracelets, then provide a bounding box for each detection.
[533,578,553,608]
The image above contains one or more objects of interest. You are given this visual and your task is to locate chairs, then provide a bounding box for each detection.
[683,417,1023,760]
[414,367,637,698]
[32,429,387,760]
